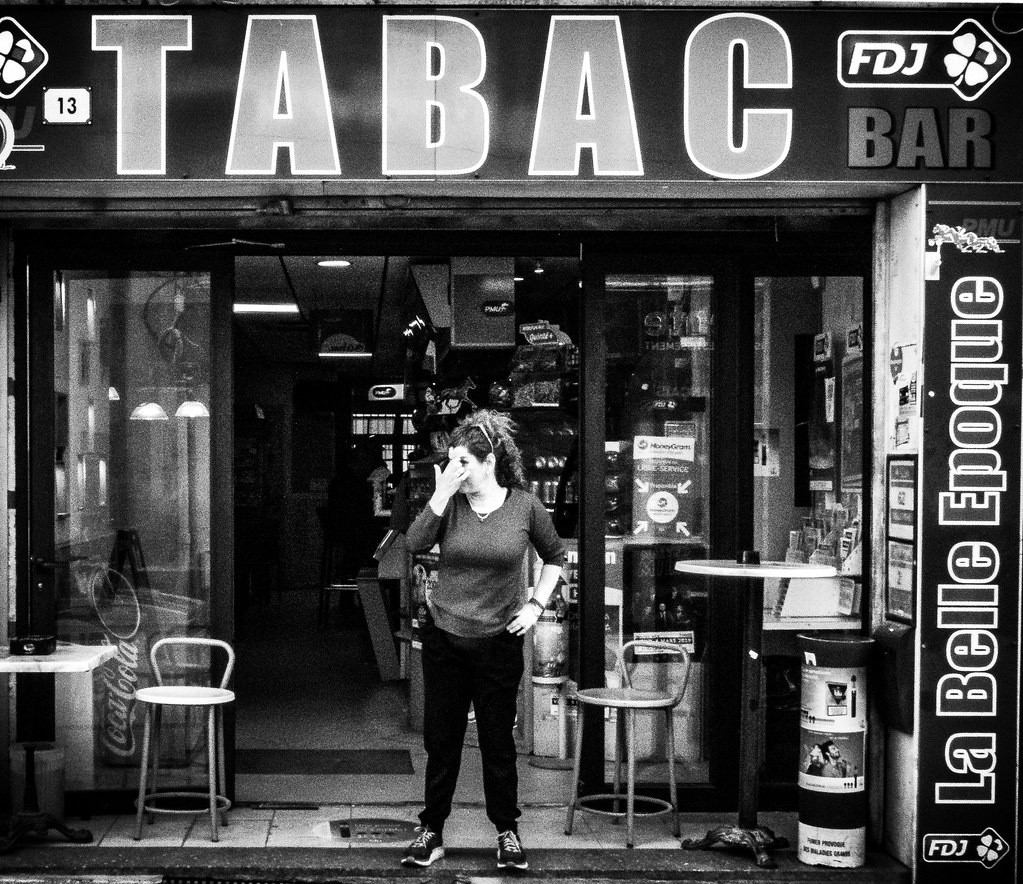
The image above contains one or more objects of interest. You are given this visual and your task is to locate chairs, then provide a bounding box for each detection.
[563,640,691,849]
[129,638,235,843]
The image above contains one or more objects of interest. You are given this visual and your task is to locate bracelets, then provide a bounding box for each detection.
[528,598,546,614]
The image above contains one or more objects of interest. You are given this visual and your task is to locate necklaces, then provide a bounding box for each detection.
[469,502,489,521]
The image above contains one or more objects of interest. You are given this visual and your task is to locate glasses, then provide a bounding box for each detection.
[470,422,494,454]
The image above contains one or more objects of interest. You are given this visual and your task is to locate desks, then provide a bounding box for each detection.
[677,558,838,867]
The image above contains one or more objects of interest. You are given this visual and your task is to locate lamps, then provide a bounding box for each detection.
[318,332,372,357]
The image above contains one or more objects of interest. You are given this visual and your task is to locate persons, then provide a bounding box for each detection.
[804,741,855,777]
[635,584,683,623]
[401,410,566,868]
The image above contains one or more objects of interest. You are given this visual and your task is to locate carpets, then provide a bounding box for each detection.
[235,748,416,775]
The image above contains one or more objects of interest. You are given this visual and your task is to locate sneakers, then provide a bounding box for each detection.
[400,824,445,866]
[496,829,528,869]
